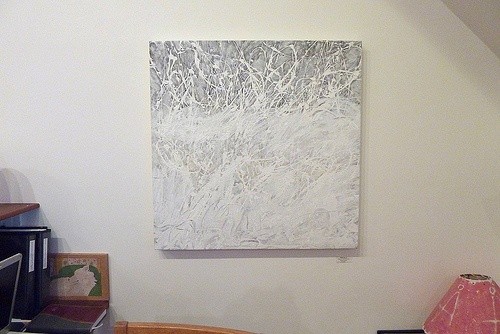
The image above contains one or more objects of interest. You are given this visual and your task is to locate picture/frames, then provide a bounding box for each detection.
[148,40,362,249]
[43,252,111,301]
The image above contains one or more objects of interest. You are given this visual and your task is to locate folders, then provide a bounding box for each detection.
[1,226,39,319]
[4,227,50,309]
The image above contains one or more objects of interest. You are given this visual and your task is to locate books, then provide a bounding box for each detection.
[25,300,108,334]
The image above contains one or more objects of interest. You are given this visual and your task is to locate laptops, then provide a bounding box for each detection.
[0,253,31,334]
